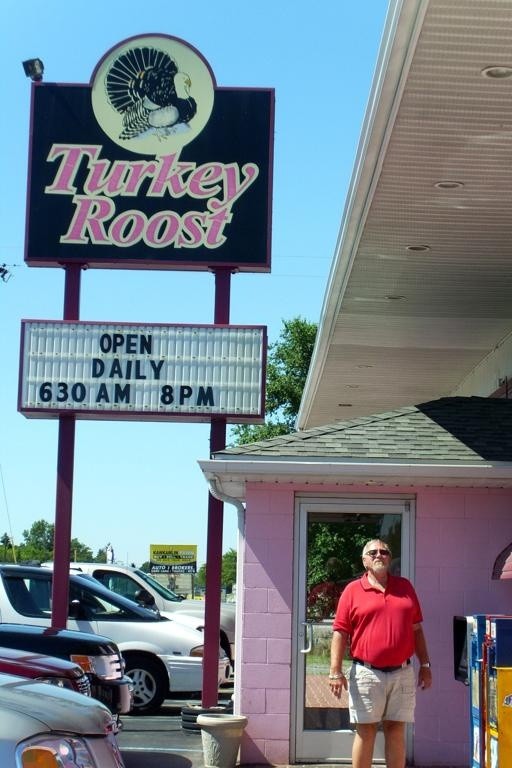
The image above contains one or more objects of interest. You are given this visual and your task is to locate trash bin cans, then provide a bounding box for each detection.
[197,714,248,768]
[221,587,227,602]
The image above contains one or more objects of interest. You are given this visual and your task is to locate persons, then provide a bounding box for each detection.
[328,539,432,768]
[308,557,354,623]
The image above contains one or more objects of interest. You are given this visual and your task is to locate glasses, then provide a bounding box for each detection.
[367,549,391,555]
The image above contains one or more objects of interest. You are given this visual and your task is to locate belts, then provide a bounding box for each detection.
[353,659,409,672]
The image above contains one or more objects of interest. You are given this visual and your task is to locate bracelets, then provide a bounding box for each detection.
[329,674,344,680]
[421,662,431,668]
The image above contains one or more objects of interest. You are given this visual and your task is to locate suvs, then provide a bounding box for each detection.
[41,561,237,665]
[0,559,232,713]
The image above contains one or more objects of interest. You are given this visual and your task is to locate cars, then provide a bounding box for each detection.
[0,646,91,698]
[0,682,125,768]
[0,622,133,715]
